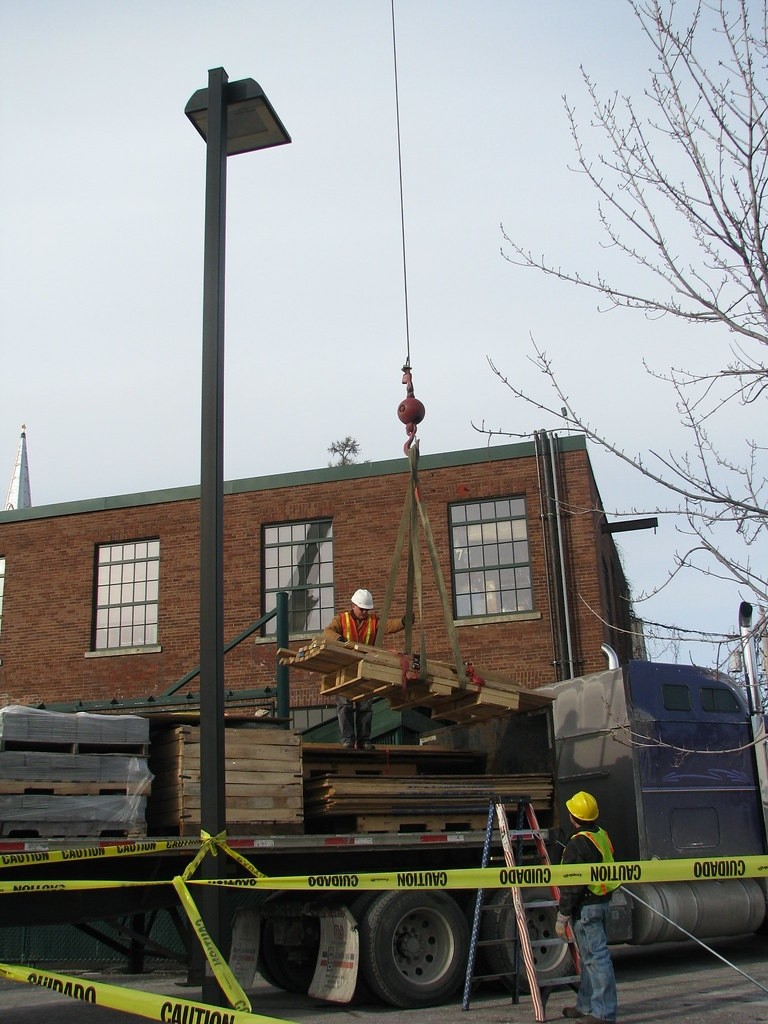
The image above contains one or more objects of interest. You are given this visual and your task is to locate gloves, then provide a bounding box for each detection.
[402,612,415,626]
[555,910,570,941]
[337,637,344,641]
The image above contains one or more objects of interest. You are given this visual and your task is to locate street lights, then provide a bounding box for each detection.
[183,65,293,1007]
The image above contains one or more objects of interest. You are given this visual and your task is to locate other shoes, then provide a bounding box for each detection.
[576,1014,616,1024]
[562,1006,586,1018]
[342,740,354,749]
[355,743,376,750]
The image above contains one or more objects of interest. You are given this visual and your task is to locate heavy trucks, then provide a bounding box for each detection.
[1,600,768,1010]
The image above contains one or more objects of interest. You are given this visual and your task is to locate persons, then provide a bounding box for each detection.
[554,791,618,1024]
[325,589,416,749]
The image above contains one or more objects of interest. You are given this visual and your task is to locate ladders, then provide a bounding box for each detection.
[461,792,581,1022]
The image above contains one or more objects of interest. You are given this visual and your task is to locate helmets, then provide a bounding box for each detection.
[566,791,599,821]
[351,589,374,609]
